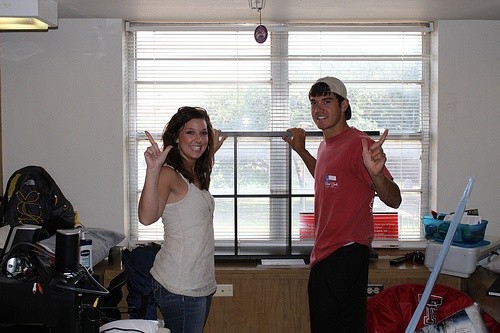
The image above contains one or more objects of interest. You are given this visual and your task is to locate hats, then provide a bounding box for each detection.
[308,77,352,120]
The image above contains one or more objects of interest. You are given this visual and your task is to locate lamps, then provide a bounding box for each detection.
[0,0,60,34]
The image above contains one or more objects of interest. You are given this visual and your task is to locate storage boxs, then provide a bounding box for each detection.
[421,213,489,246]
[424,238,500,279]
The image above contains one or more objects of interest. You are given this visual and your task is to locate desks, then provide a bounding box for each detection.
[101,259,464,333]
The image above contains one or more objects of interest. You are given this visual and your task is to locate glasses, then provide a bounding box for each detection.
[178,107,206,115]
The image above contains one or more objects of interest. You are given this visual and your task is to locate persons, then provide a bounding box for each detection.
[138,107,227,333]
[282,76,402,333]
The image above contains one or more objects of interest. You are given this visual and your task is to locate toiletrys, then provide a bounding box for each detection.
[79,238,93,271]
